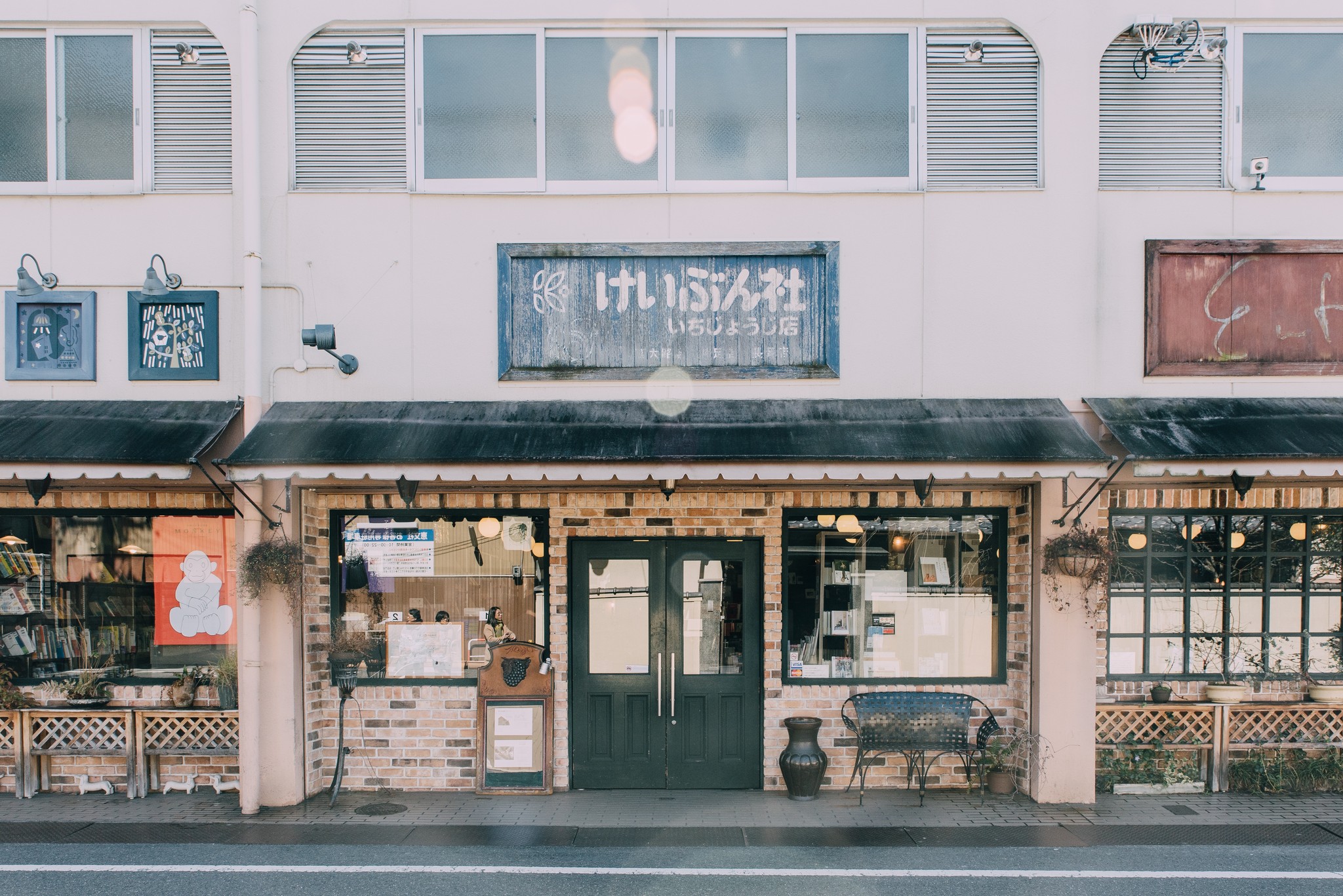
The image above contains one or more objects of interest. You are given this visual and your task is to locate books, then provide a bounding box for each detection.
[0,542,155,677]
[720,557,895,678]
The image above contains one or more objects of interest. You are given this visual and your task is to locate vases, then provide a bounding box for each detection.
[779,716,828,801]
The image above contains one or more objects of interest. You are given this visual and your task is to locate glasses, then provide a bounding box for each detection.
[494,613,503,615]
[441,617,449,620]
[407,615,414,618]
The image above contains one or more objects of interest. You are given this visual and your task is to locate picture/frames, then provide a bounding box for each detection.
[127,291,219,381]
[4,291,97,383]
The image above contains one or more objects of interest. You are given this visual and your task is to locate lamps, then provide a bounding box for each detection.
[813,514,1001,559]
[15,254,59,297]
[478,516,545,557]
[1126,516,1307,550]
[0,516,29,546]
[141,254,182,296]
[118,517,147,554]
[301,324,359,375]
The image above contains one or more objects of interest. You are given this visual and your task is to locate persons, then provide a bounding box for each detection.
[483,606,517,667]
[399,609,426,678]
[426,611,452,678]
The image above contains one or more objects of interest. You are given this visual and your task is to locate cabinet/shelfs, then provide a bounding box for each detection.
[813,531,864,678]
[0,537,225,685]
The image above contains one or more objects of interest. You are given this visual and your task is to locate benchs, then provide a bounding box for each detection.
[841,691,1002,808]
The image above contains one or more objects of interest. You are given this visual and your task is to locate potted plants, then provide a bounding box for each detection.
[1096,720,1343,796]
[0,601,238,709]
[340,526,389,623]
[310,613,383,696]
[231,527,315,628]
[1032,524,1115,631]
[967,735,1022,798]
[1150,592,1343,703]
